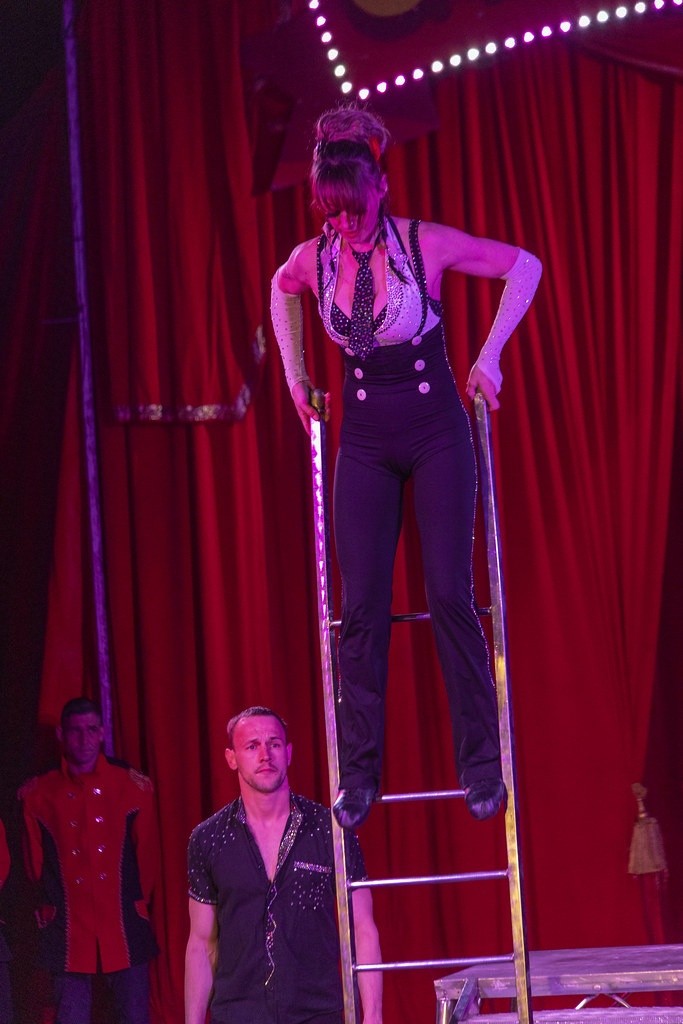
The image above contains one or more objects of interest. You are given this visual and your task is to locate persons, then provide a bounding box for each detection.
[184,707,383,1024]
[0,697,159,1024]
[271,102,542,828]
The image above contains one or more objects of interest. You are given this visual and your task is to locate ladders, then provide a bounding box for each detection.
[303,385,534,1024]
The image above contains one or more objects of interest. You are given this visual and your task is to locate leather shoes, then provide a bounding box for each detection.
[332,783,383,829]
[463,780,505,819]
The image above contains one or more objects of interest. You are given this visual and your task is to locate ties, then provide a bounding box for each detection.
[349,252,375,361]
